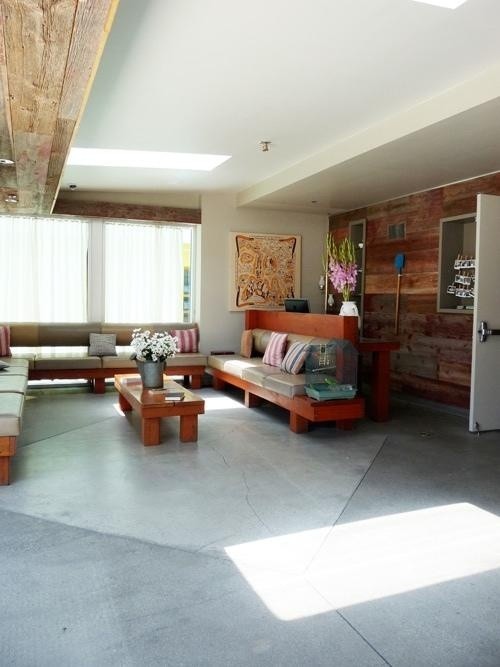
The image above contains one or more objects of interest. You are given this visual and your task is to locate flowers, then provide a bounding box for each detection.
[322,231,358,302]
[127,327,180,363]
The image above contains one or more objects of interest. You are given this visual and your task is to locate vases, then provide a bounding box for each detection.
[130,353,168,389]
[339,301,360,328]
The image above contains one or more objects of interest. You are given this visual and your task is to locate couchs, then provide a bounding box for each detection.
[2,323,207,395]
[205,309,365,433]
[0,326,29,485]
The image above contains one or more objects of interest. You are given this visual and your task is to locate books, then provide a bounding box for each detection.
[164,392,185,401]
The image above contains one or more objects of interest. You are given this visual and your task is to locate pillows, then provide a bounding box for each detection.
[89,332,117,356]
[0,360,11,370]
[0,325,12,357]
[278,341,313,375]
[170,329,197,352]
[240,329,252,358]
[263,331,289,367]
